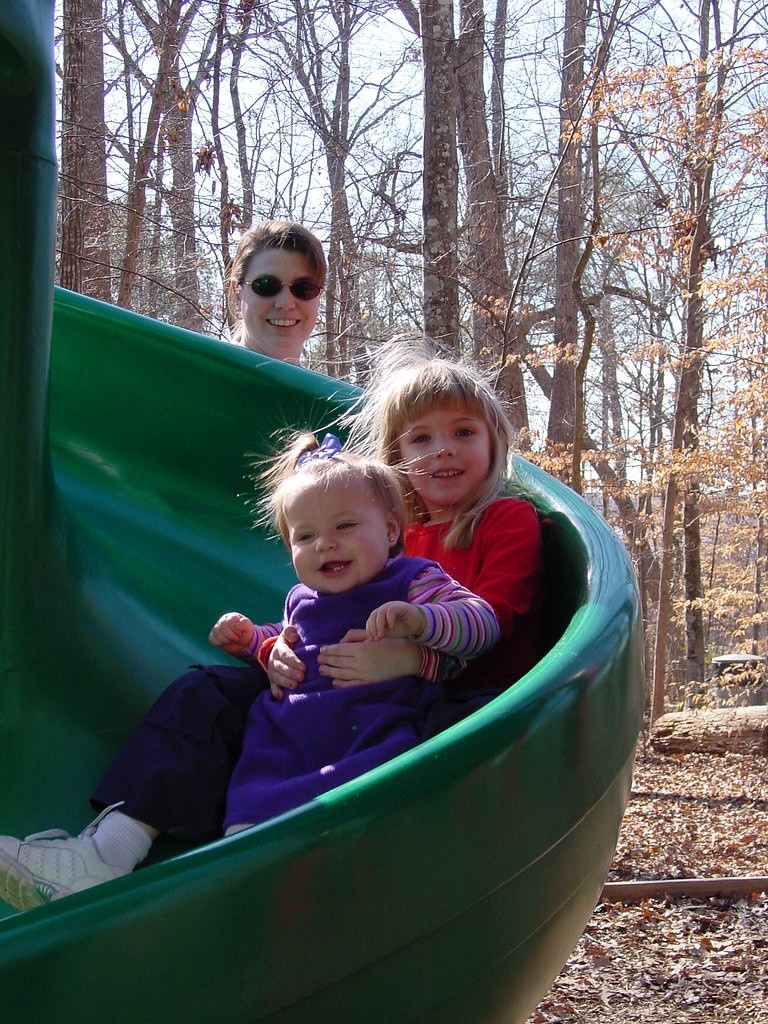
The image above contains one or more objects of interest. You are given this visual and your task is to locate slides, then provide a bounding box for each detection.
[0,284,647,1024]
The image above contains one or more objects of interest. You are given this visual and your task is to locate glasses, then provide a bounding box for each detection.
[243,277,324,300]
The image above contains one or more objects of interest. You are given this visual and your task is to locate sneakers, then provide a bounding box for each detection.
[0,801,134,911]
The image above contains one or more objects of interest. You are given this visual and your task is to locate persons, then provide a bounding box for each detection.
[0,355,540,911]
[226,221,328,372]
[209,431,499,839]
